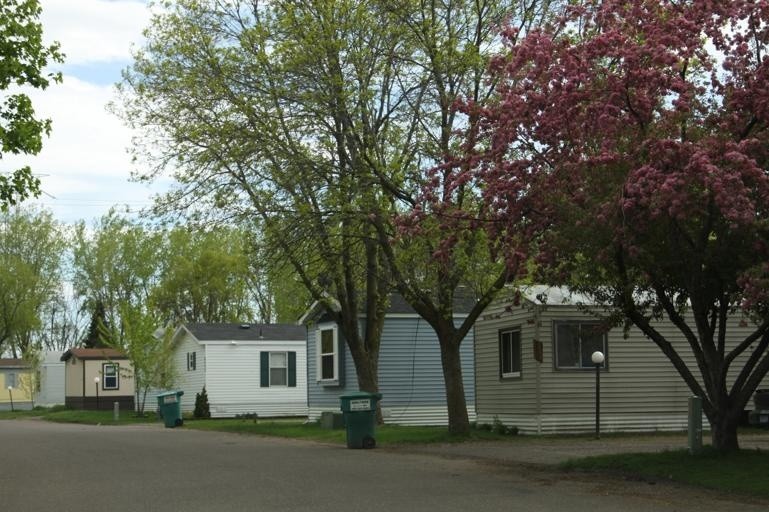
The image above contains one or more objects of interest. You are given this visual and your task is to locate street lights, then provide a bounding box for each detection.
[591,351,605,440]
[94,377,100,410]
[8,386,14,412]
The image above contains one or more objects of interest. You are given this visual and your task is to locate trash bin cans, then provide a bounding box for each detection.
[338,393,383,449]
[156,391,184,427]
